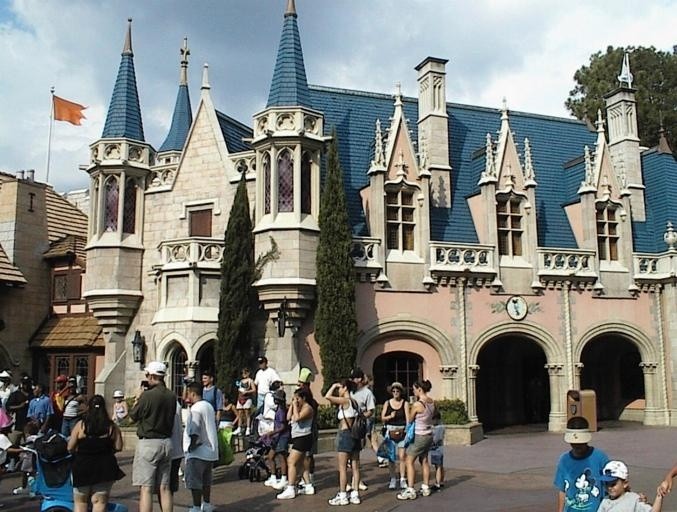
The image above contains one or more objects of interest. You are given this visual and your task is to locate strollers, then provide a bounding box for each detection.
[237,431,281,482]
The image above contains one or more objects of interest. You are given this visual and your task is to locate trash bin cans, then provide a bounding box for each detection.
[566,390,596,432]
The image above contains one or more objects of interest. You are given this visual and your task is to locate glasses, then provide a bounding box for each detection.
[392,391,400,393]
[53,379,61,383]
[146,374,149,378]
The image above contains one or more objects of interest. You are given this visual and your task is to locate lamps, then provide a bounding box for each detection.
[131,330,147,371]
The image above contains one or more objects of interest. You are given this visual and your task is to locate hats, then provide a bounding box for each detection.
[564,428,592,445]
[346,368,364,378]
[599,461,628,483]
[0,370,13,380]
[143,362,167,376]
[387,382,407,396]
[113,391,124,398]
[272,390,286,402]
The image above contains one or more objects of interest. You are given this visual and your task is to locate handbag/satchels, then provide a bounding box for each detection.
[389,429,404,441]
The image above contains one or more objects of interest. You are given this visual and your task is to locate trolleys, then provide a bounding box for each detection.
[19,432,128,512]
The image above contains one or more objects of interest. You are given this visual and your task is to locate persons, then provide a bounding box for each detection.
[0,356,446,511]
[595,458,668,512]
[655,463,676,498]
[552,415,611,512]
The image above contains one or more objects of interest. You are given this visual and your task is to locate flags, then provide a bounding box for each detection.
[53,96,89,127]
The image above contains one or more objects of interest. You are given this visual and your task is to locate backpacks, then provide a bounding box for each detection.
[341,398,366,447]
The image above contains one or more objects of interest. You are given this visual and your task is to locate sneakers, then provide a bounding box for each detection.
[12,487,29,494]
[245,428,251,436]
[387,478,444,501]
[328,481,368,506]
[232,427,241,436]
[200,503,212,512]
[264,474,315,500]
